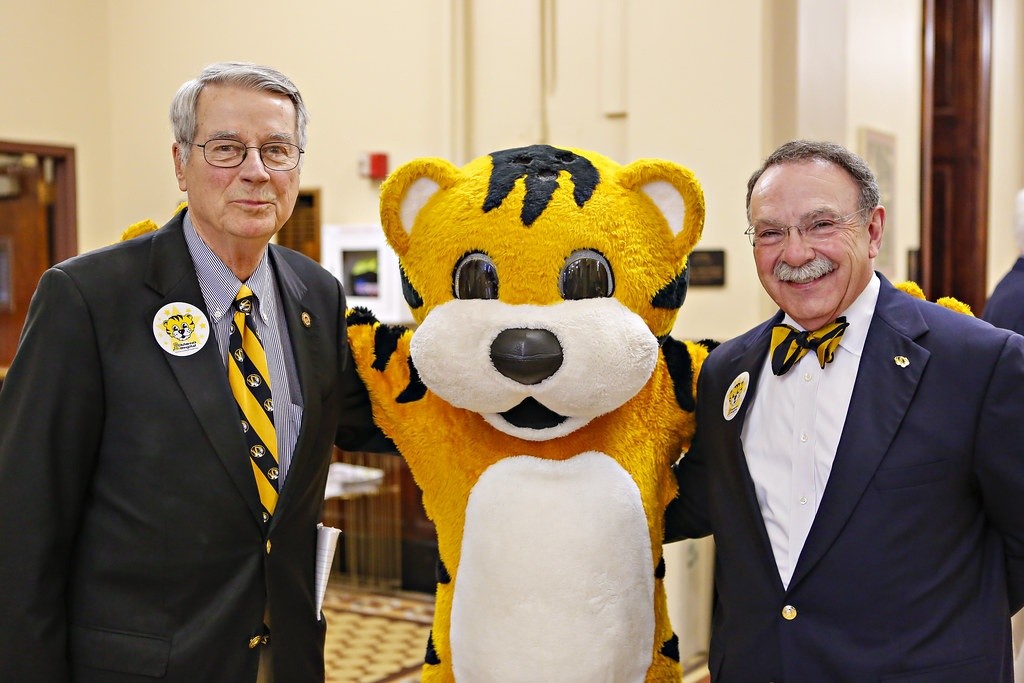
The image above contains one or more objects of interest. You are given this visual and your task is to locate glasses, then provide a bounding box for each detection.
[744,207,868,251]
[184,139,304,171]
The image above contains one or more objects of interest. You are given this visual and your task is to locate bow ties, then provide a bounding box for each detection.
[771,316,850,376]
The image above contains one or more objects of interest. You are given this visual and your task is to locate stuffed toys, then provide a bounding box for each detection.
[118,147,974,682]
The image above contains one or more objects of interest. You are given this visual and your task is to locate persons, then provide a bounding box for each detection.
[1,61,399,683]
[663,140,1024,683]
[982,188,1024,335]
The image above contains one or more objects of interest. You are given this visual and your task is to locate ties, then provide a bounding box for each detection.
[228,284,280,646]
